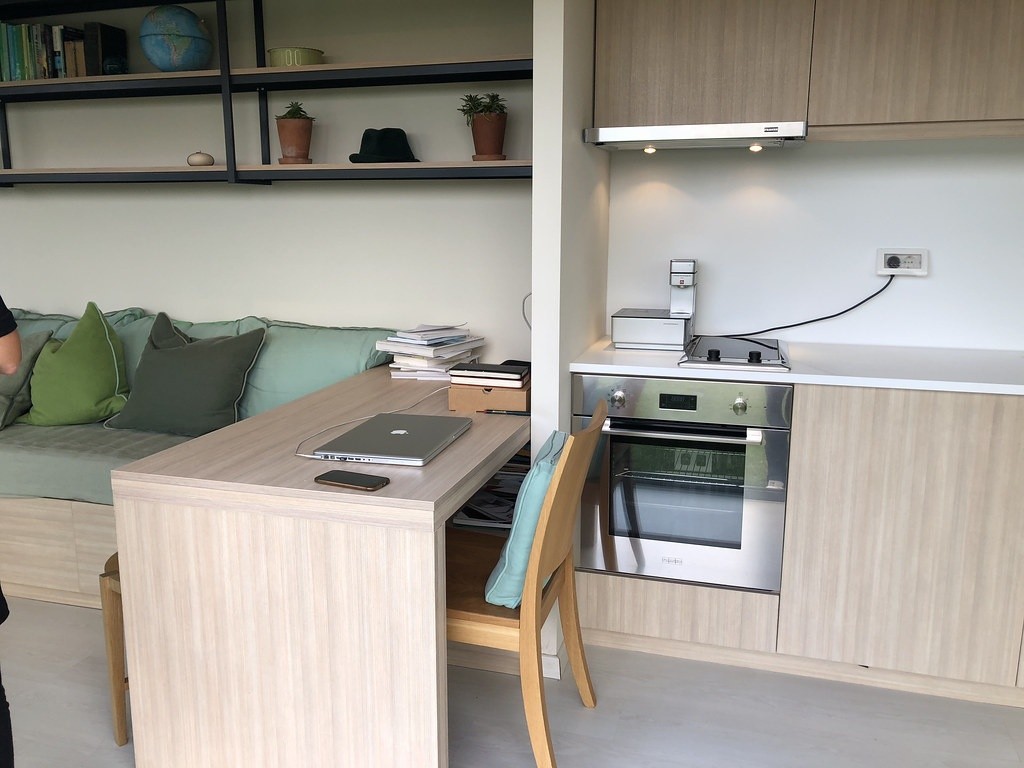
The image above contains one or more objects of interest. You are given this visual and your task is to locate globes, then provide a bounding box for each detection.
[138,5,214,71]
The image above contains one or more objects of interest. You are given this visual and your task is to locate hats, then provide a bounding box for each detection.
[349,127,421,163]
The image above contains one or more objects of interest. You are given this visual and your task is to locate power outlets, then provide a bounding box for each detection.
[878,248,929,276]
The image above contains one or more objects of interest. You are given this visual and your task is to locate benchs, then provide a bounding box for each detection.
[0,308,400,608]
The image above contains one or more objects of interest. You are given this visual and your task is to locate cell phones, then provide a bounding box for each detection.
[314,470,390,492]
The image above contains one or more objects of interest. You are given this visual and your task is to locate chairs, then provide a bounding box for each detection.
[444,399,609,768]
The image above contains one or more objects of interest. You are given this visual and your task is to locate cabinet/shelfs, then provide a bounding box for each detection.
[582,0,1024,143]
[778,344,1024,708]
[0,0,534,188]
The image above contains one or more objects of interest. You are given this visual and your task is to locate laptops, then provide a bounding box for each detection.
[313,414,472,467]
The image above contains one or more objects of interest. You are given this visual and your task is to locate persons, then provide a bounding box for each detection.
[0,296,22,768]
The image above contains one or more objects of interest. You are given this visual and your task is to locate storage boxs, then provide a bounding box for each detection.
[448,387,529,412]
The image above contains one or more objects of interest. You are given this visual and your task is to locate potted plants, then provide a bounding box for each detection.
[456,93,507,160]
[275,101,316,164]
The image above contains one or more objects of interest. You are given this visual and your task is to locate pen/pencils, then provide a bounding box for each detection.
[484,409,533,416]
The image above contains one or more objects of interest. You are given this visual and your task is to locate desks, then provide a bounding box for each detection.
[110,363,530,768]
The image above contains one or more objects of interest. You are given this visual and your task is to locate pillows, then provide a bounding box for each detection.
[0,330,55,431]
[13,301,130,427]
[102,312,265,437]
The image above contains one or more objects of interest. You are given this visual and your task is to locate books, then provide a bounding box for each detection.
[375,321,486,381]
[0,21,129,82]
[448,362,530,389]
